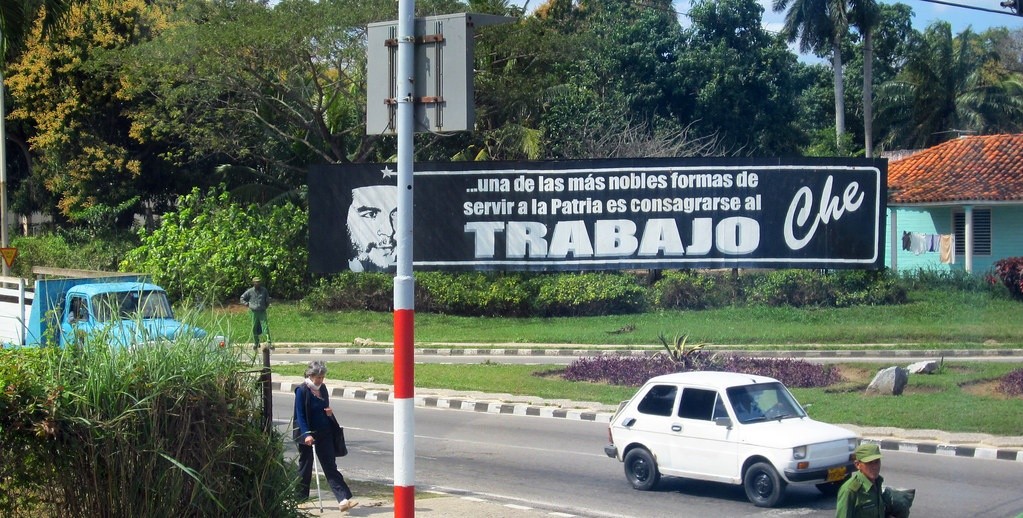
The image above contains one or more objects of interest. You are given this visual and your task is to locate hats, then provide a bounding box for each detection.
[855,443,882,463]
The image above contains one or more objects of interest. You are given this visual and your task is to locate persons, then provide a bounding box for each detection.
[240,276,276,350]
[291,359,358,513]
[835,443,885,518]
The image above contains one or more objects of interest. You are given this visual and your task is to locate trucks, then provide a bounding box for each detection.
[0,272,228,363]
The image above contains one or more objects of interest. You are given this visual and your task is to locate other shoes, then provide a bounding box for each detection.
[339,500,359,512]
[297,503,307,512]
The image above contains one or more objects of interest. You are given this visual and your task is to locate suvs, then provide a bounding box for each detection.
[604,371,861,509]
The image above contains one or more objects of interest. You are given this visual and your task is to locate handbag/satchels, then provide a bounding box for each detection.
[329,412,348,457]
